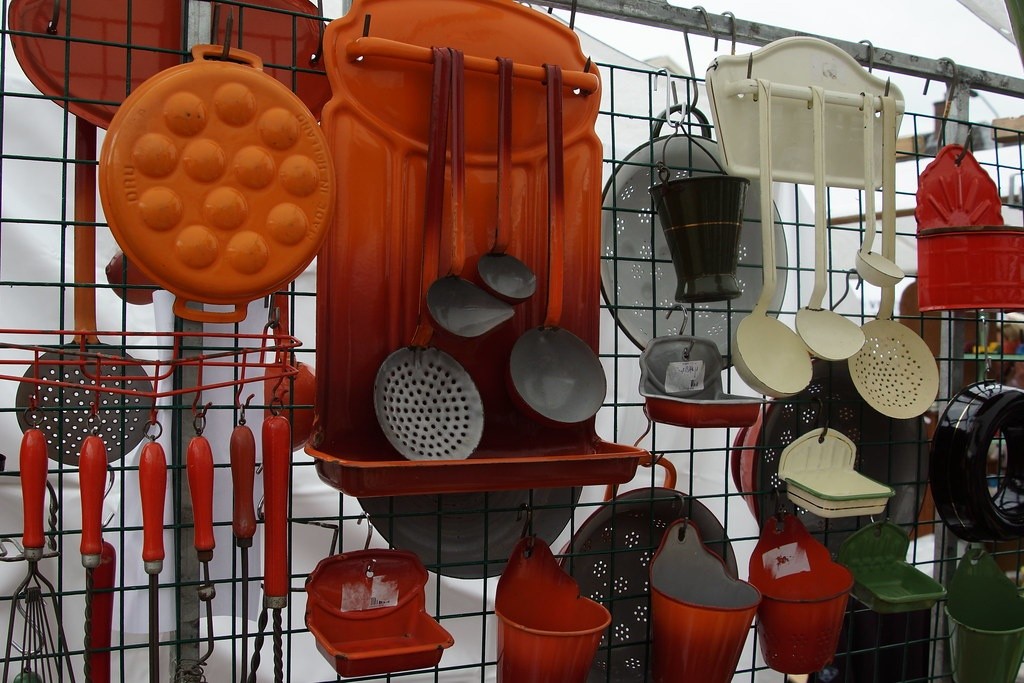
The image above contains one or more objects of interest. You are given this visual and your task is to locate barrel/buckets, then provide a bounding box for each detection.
[918,145,1024,312]
[944,547,1024,682]
[649,122,752,302]
[748,514,853,675]
[646,518,761,682]
[495,537,611,683]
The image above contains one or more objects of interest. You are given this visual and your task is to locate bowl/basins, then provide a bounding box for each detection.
[594,103,790,371]
